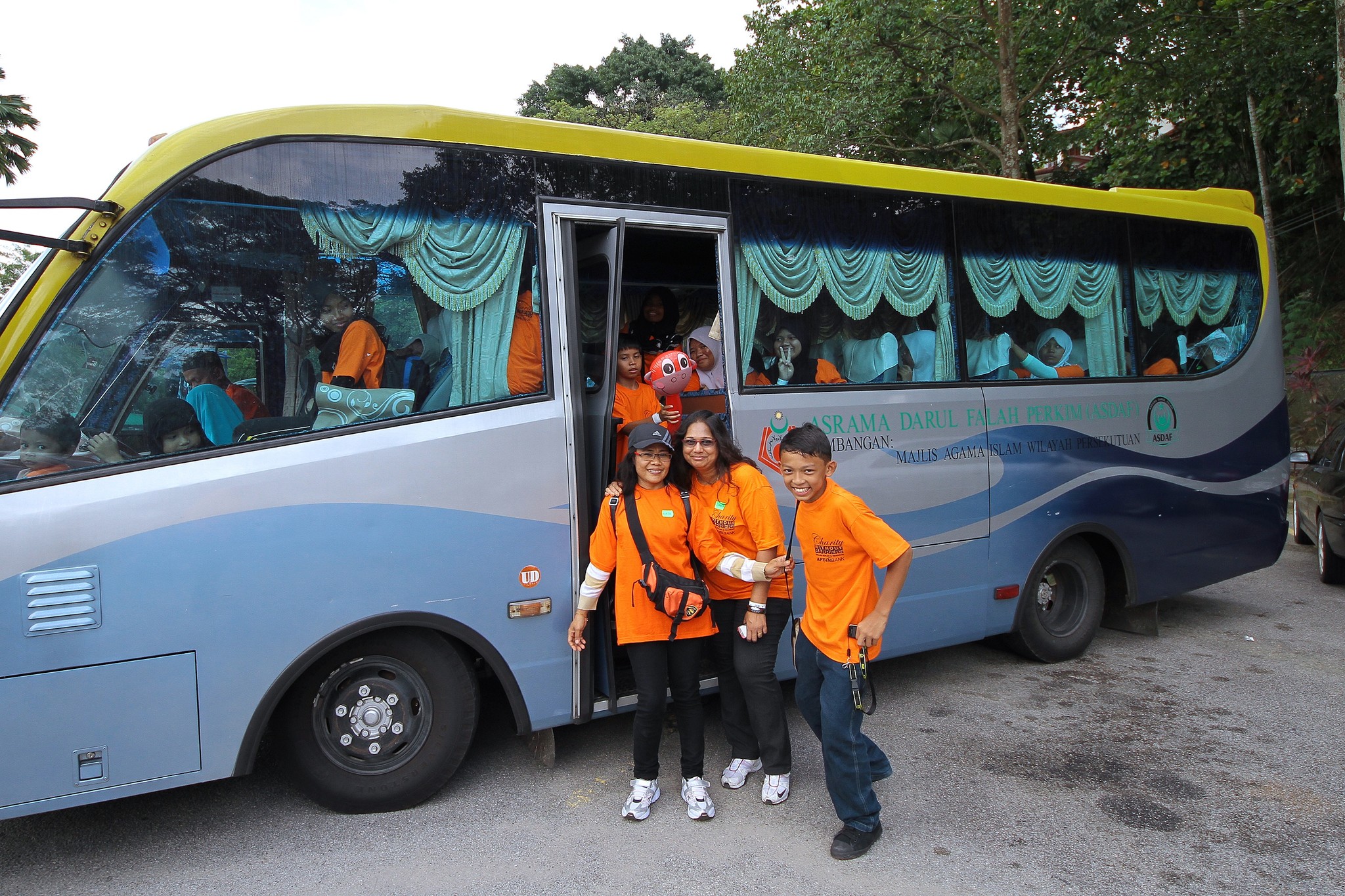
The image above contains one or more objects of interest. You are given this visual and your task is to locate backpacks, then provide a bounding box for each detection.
[342,319,430,413]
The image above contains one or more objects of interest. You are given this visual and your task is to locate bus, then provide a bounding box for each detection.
[0,102,1297,825]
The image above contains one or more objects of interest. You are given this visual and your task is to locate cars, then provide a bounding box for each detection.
[1289,421,1345,586]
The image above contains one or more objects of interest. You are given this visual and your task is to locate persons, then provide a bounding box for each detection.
[87,397,209,463]
[991,328,1085,379]
[20,410,81,477]
[620,286,683,383]
[182,350,272,420]
[684,326,772,391]
[310,272,386,389]
[611,333,681,472]
[1193,324,1217,372]
[754,317,847,386]
[604,410,794,805]
[402,334,440,363]
[778,423,912,859]
[1138,325,1182,375]
[507,263,544,394]
[568,422,795,821]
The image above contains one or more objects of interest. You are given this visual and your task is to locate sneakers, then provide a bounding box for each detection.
[681,776,715,821]
[831,818,882,860]
[761,770,790,805]
[721,756,762,789]
[622,778,660,821]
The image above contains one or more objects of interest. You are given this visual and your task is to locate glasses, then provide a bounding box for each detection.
[681,439,717,448]
[635,452,672,461]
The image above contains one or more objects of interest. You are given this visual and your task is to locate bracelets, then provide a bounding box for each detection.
[652,412,662,424]
[777,378,789,385]
[746,601,766,614]
[575,612,588,621]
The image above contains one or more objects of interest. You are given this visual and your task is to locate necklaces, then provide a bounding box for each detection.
[696,466,720,482]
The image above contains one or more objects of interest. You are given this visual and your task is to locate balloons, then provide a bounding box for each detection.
[644,350,697,432]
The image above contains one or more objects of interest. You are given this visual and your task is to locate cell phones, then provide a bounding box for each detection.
[848,624,865,641]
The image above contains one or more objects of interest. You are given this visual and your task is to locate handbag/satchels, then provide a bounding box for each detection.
[638,561,709,622]
[791,617,803,672]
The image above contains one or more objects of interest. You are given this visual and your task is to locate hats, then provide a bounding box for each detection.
[183,351,223,371]
[628,423,674,452]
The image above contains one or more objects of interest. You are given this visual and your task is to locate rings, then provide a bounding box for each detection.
[758,633,762,637]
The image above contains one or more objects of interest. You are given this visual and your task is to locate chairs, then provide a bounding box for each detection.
[764,334,1087,382]
[420,308,453,411]
